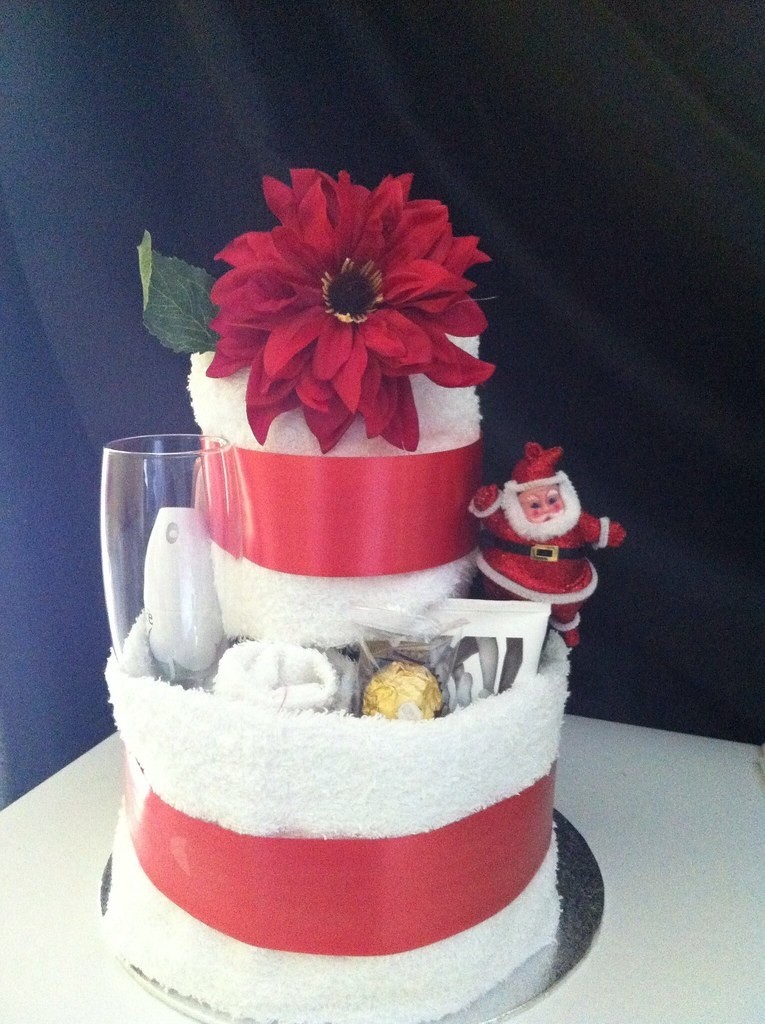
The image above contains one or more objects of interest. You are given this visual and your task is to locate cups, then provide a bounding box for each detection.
[100,436,257,686]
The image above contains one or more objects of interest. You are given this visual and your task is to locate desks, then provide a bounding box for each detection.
[0,715,765,1024]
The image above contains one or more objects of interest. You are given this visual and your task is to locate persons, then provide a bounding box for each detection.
[465,441,627,646]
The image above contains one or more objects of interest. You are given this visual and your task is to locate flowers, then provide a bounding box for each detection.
[138,168,497,455]
